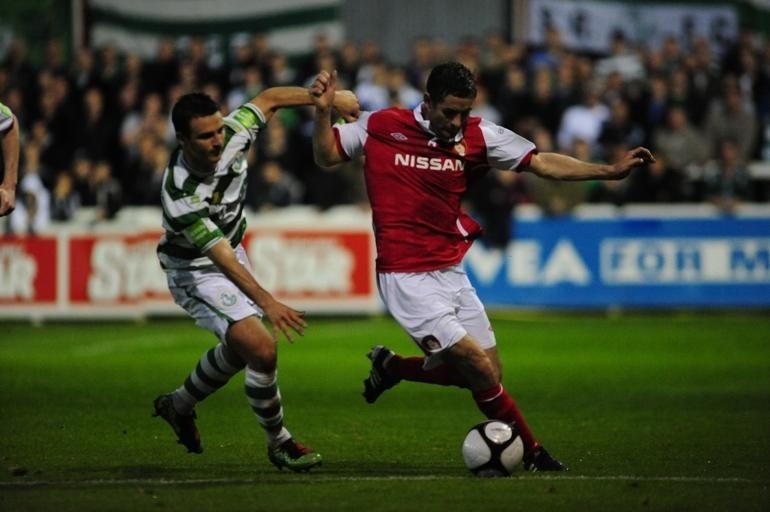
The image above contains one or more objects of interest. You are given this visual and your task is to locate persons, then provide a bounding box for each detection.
[309,59,657,472]
[1,12,769,216]
[153,85,360,472]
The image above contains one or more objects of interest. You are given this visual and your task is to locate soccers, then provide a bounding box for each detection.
[462,419,524,477]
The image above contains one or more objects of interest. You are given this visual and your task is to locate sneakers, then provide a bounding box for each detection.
[362,345,400,403]
[153,394,201,454]
[523,447,564,471]
[268,437,322,472]
